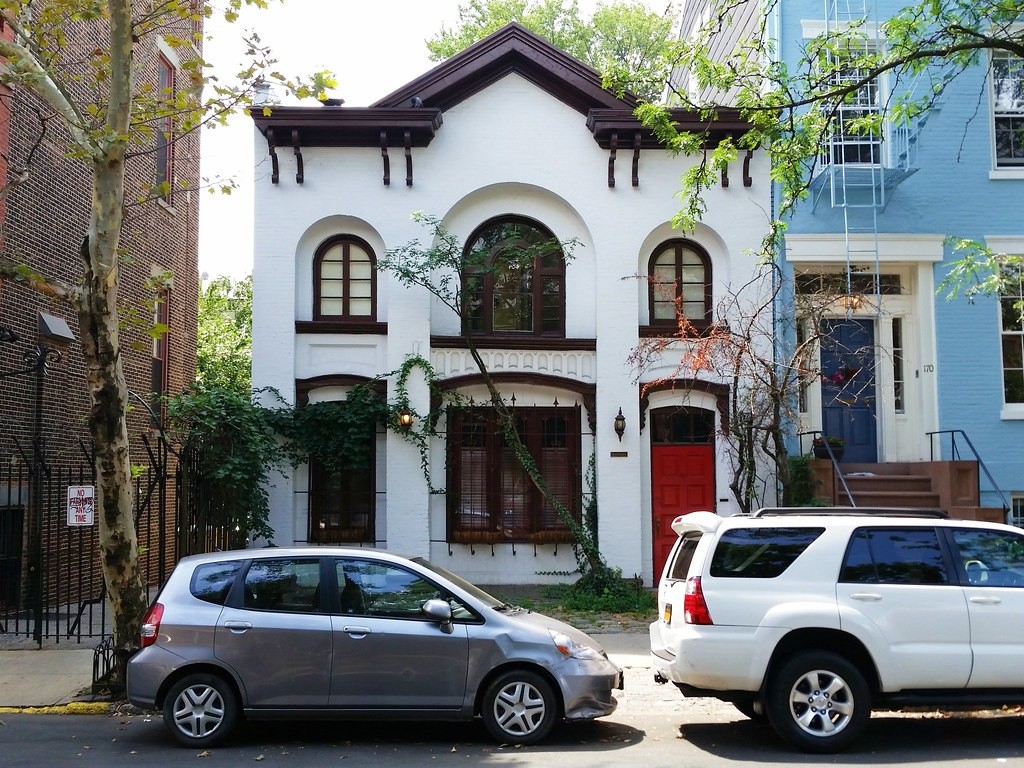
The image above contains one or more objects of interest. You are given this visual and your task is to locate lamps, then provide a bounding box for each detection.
[399,405,414,438]
[614,407,626,443]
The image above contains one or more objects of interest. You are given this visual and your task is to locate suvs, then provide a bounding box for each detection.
[648,507,1024,754]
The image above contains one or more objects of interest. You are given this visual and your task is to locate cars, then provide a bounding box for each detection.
[125,546,623,749]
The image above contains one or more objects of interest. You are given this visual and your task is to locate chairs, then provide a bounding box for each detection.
[343,573,368,615]
